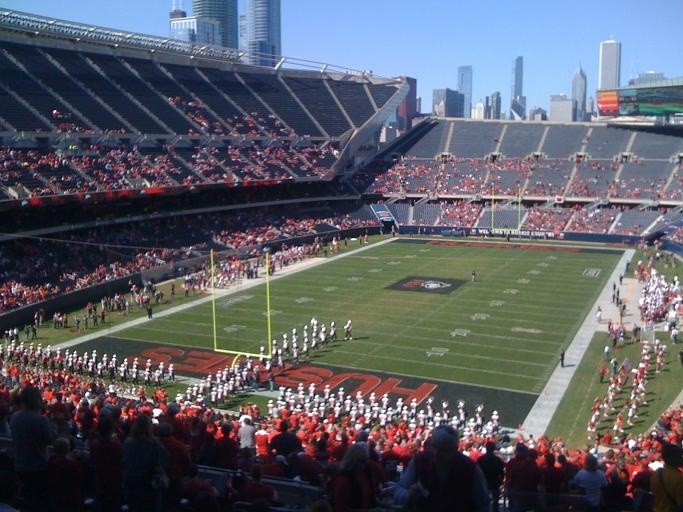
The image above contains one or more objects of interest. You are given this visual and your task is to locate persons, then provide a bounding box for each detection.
[1,134,369,351]
[595,245,681,349]
[1,350,682,512]
[469,268,477,282]
[370,154,682,245]
[35,96,296,137]
